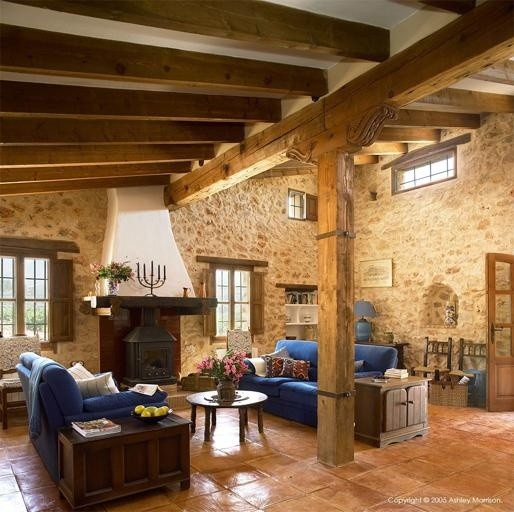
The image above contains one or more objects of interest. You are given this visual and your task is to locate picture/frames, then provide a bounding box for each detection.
[357,258,394,288]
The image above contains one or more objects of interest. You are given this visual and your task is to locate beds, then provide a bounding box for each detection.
[186,390,268,442]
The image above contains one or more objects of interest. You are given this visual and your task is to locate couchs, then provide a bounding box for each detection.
[239,340,398,428]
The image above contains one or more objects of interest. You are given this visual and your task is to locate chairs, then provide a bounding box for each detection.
[447,338,491,382]
[411,336,453,379]
[0,335,42,429]
[15,350,170,484]
[227,329,253,358]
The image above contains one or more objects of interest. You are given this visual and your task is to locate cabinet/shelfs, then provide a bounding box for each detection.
[56,413,193,510]
[285,290,318,342]
[354,376,432,449]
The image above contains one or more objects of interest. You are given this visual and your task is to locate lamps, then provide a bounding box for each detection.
[354,300,376,342]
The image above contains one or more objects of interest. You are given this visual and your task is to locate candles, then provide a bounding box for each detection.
[137,259,166,279]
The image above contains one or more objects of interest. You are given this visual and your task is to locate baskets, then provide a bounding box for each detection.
[428,374,468,407]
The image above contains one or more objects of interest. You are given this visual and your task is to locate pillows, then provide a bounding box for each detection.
[265,355,284,377]
[65,361,94,380]
[279,357,310,381]
[260,346,291,359]
[76,371,121,398]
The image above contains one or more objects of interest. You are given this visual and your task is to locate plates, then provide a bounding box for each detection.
[129,409,173,424]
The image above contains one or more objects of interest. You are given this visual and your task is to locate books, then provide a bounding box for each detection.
[128,383,164,396]
[72,417,121,439]
[371,367,409,383]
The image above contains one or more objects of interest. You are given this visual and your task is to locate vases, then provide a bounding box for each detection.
[110,280,119,296]
[94,278,111,297]
[216,379,237,401]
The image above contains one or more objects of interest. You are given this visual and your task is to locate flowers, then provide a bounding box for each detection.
[197,349,248,381]
[90,260,137,283]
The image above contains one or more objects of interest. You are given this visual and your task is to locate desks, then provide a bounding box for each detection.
[356,341,410,369]
